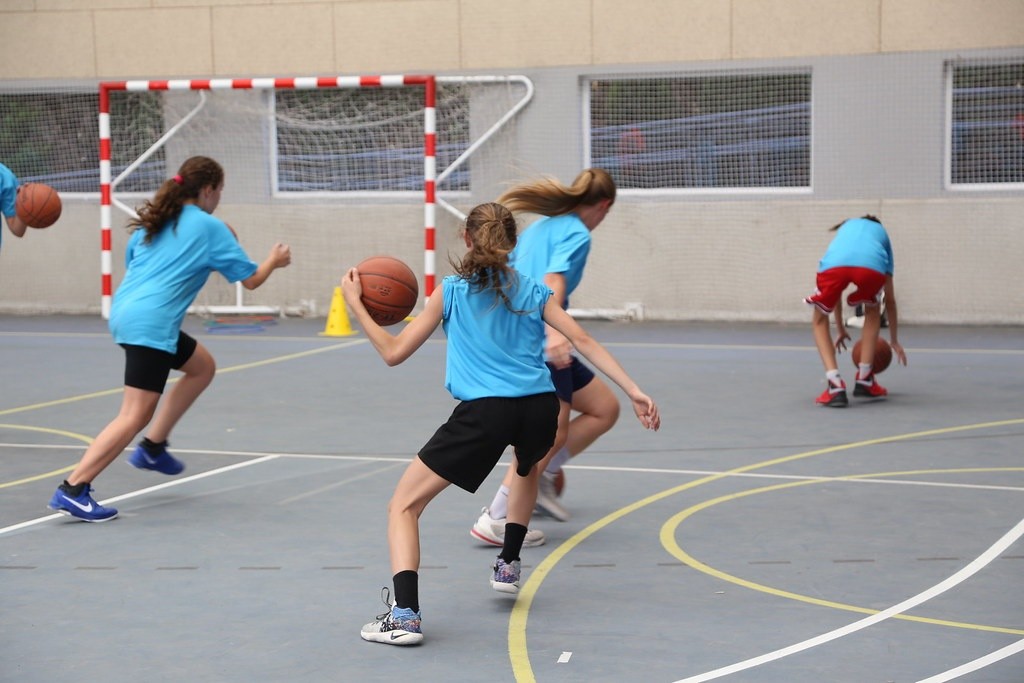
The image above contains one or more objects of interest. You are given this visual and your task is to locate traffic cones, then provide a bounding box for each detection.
[319,287,361,337]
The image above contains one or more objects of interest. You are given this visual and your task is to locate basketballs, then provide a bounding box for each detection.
[852,335,893,374]
[350,255,420,326]
[16,184,63,229]
[212,220,240,273]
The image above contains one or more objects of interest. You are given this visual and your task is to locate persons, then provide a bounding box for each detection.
[803,214,907,406]
[0,162,35,251]
[341,202,660,644]
[47,156,291,523]
[470,166,620,548]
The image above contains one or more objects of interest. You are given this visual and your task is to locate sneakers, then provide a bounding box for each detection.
[46,480,119,523]
[853,371,887,397]
[816,379,848,406]
[361,586,423,644]
[470,506,546,548]
[536,473,568,522]
[127,444,184,475]
[489,549,520,594]
